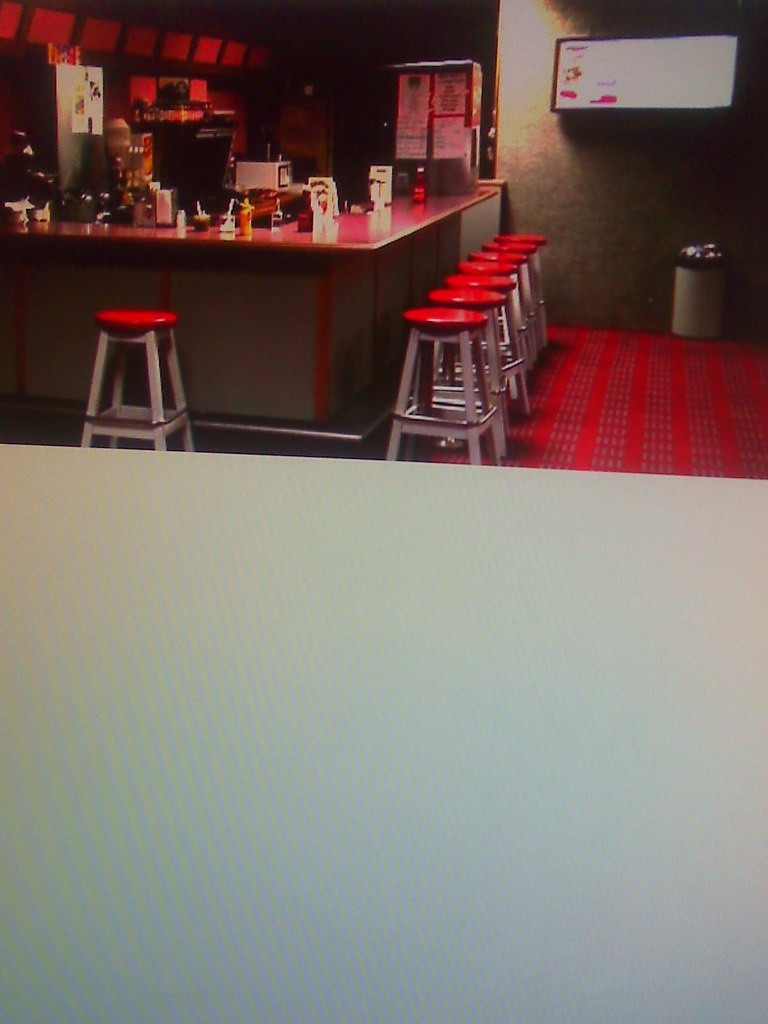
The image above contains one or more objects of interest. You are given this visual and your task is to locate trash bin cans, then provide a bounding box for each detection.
[671,241,732,339]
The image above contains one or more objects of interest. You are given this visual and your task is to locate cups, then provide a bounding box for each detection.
[219,215,235,231]
[271,212,283,233]
[194,215,211,232]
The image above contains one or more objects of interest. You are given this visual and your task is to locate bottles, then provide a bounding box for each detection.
[176,209,188,235]
[239,197,253,236]
[412,167,426,204]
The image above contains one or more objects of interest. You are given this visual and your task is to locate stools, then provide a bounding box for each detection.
[387,235,548,467]
[80,308,195,452]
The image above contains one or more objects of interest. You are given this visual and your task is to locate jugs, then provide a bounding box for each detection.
[129,98,154,131]
